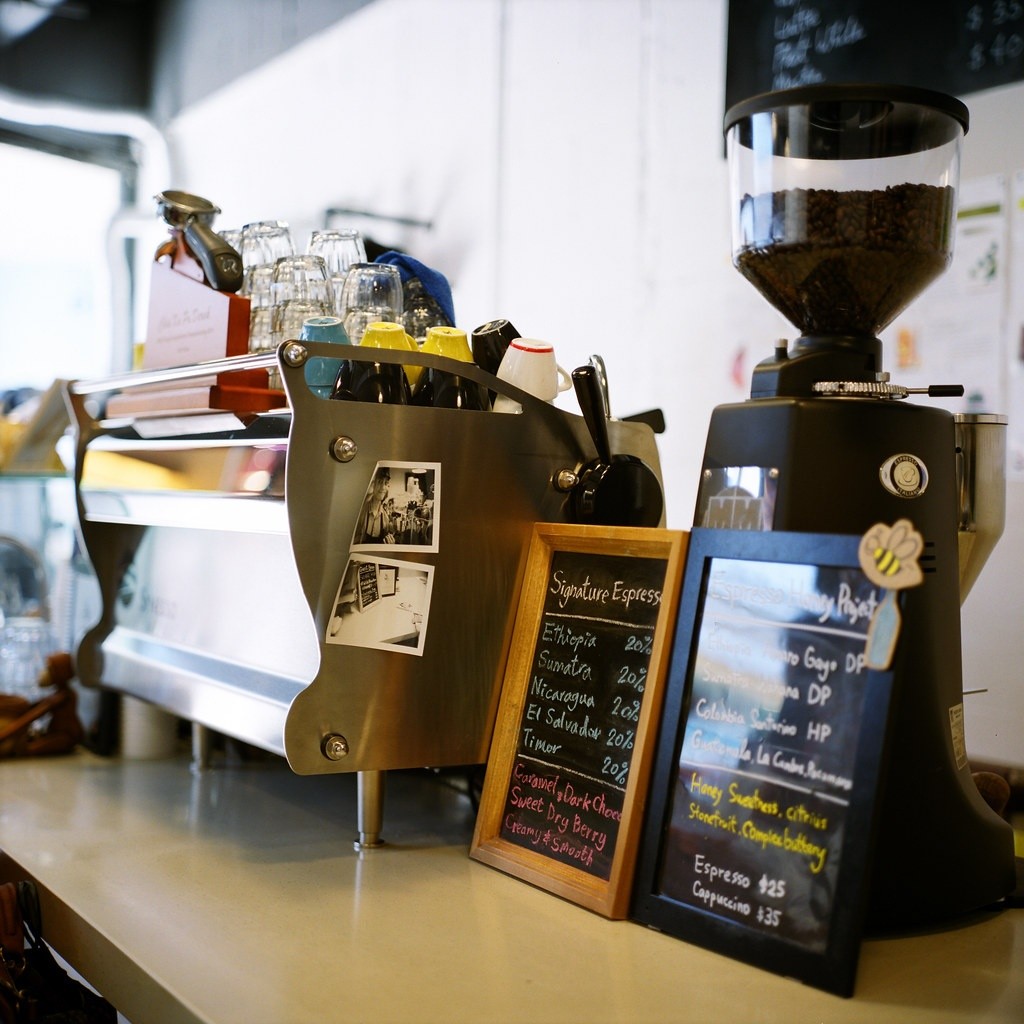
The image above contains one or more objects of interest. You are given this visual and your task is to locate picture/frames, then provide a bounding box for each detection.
[468,522,690,920]
[626,519,924,999]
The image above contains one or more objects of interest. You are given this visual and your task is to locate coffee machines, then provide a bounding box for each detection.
[692,78,1016,922]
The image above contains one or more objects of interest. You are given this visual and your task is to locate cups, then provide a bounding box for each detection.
[0,611,78,698]
[217,219,569,413]
[124,697,183,757]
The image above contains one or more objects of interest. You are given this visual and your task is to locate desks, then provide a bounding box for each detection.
[0,754,1023,1024]
